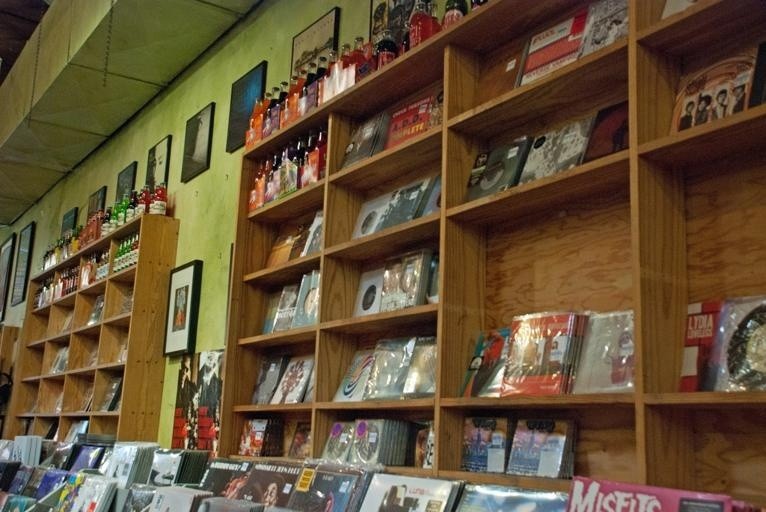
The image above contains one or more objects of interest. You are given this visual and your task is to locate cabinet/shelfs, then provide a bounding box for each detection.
[0,324,22,419]
[0,214,183,443]
[220,1,765,512]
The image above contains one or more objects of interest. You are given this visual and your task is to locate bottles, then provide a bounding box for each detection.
[242,0,487,149]
[59,265,80,295]
[112,232,140,273]
[43,209,102,272]
[247,125,328,211]
[87,248,110,280]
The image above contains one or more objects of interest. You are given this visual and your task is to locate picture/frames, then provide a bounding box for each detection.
[145,135,172,188]
[0,234,18,322]
[86,185,108,220]
[60,207,78,239]
[10,219,38,307]
[113,161,138,205]
[225,61,268,154]
[369,1,430,45]
[180,101,215,183]
[291,5,341,83]
[161,257,204,357]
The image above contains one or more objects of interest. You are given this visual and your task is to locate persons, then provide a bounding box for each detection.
[174,286,187,327]
[182,117,204,180]
[175,350,225,417]
[152,138,167,190]
[678,84,745,133]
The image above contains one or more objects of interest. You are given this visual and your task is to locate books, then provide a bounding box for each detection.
[354,247,442,317]
[460,419,579,480]
[351,173,441,242]
[250,352,314,405]
[0,438,756,510]
[23,284,133,438]
[239,416,312,459]
[677,295,766,393]
[261,268,321,334]
[466,311,634,399]
[321,420,435,469]
[330,334,435,405]
[464,100,629,205]
[514,0,628,91]
[263,211,323,267]
[338,95,444,169]
